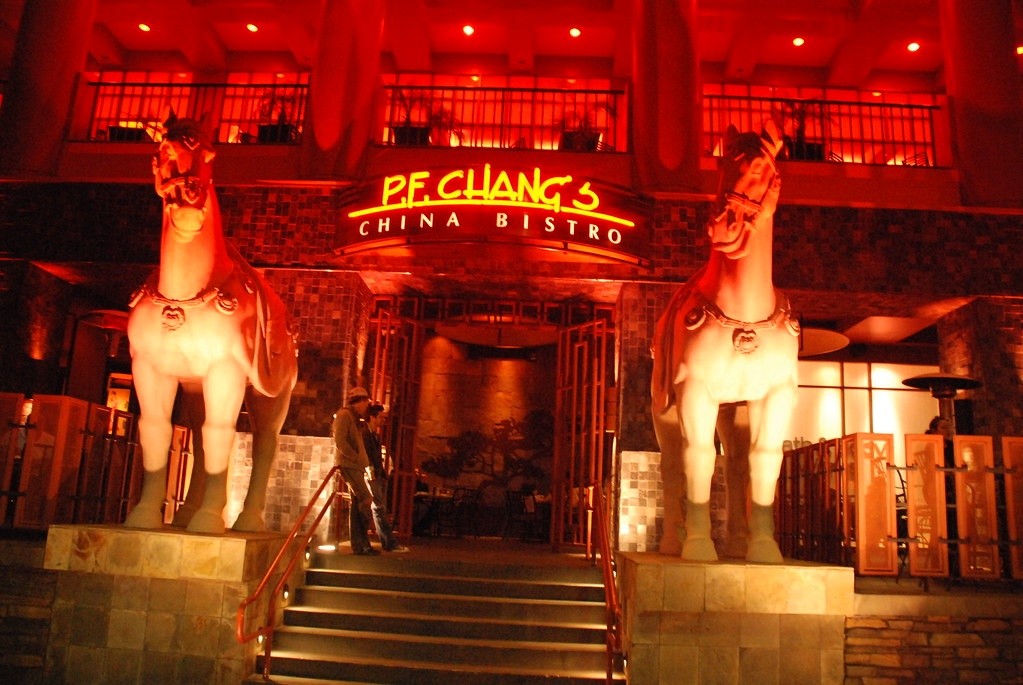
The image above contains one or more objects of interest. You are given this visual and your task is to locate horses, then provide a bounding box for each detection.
[650,124,802,562]
[120,109,302,534]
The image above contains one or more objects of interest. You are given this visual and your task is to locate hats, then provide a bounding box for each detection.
[349,387,368,398]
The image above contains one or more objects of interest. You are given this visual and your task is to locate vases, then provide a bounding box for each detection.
[105,125,145,142]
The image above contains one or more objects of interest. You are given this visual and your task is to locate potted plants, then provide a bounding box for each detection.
[257,93,299,144]
[555,102,615,151]
[781,98,833,160]
[393,92,464,145]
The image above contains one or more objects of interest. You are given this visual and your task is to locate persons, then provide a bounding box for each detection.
[924,417,958,555]
[333,386,410,554]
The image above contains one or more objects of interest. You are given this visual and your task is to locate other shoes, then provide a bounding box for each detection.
[386,544,409,553]
[358,548,381,555]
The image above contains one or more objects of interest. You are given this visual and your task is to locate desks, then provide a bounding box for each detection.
[533,495,549,539]
[896,503,956,584]
[414,491,453,535]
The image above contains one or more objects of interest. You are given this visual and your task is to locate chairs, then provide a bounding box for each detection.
[889,467,960,593]
[501,488,547,543]
[416,485,482,540]
